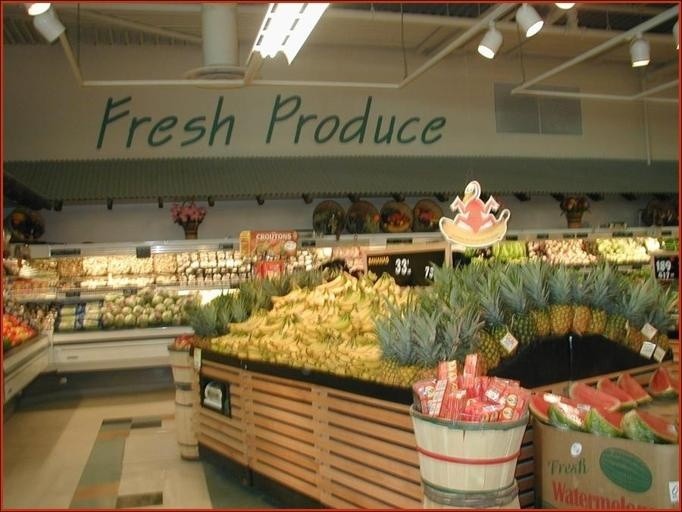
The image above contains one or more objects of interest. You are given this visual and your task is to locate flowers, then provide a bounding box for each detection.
[171,202,206,225]
[560,197,592,214]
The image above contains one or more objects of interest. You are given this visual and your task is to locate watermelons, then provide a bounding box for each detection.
[599,448,652,492]
[527,364,678,444]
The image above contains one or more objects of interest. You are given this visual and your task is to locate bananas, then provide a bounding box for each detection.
[209,272,424,381]
[441,210,508,246]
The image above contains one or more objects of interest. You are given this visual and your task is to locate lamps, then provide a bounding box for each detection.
[246,3,331,66]
[478,2,576,59]
[24,3,66,44]
[629,19,678,68]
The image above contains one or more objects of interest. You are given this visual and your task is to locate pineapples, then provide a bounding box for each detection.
[373,252,679,388]
[183,269,321,350]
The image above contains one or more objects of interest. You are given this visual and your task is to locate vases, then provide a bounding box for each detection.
[566,212,583,228]
[179,221,202,239]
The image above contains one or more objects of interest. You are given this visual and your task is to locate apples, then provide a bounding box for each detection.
[3,313,35,345]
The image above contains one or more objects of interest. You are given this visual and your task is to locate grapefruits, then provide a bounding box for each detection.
[101,287,194,328]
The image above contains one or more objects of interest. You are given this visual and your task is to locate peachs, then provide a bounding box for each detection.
[564,198,588,210]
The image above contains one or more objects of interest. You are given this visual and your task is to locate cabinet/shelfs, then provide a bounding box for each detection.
[3,278,240,405]
